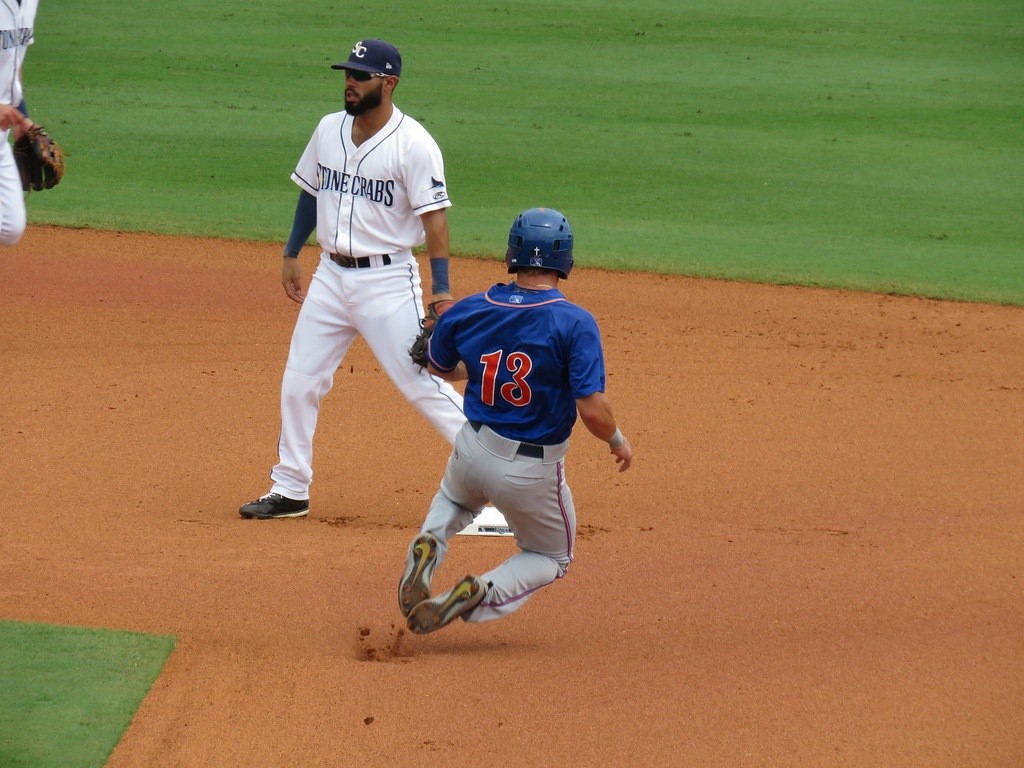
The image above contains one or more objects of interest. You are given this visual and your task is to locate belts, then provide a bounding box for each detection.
[330,252,391,268]
[468,419,544,459]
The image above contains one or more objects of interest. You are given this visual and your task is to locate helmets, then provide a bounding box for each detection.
[505,206,573,279]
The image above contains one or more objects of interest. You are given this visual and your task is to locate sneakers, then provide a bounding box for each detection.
[407,575,486,634]
[239,492,309,518]
[397,533,439,618]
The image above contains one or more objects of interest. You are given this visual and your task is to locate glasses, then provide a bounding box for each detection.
[345,68,391,81]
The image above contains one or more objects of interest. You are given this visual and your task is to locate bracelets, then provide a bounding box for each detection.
[607,427,623,450]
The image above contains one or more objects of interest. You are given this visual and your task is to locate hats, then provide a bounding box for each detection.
[331,39,402,77]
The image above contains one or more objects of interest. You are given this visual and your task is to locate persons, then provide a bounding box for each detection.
[238,39,467,519]
[398,207,632,634]
[0,0,48,246]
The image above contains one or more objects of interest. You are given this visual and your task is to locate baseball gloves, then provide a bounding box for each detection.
[407,299,459,375]
[13,122,65,202]
